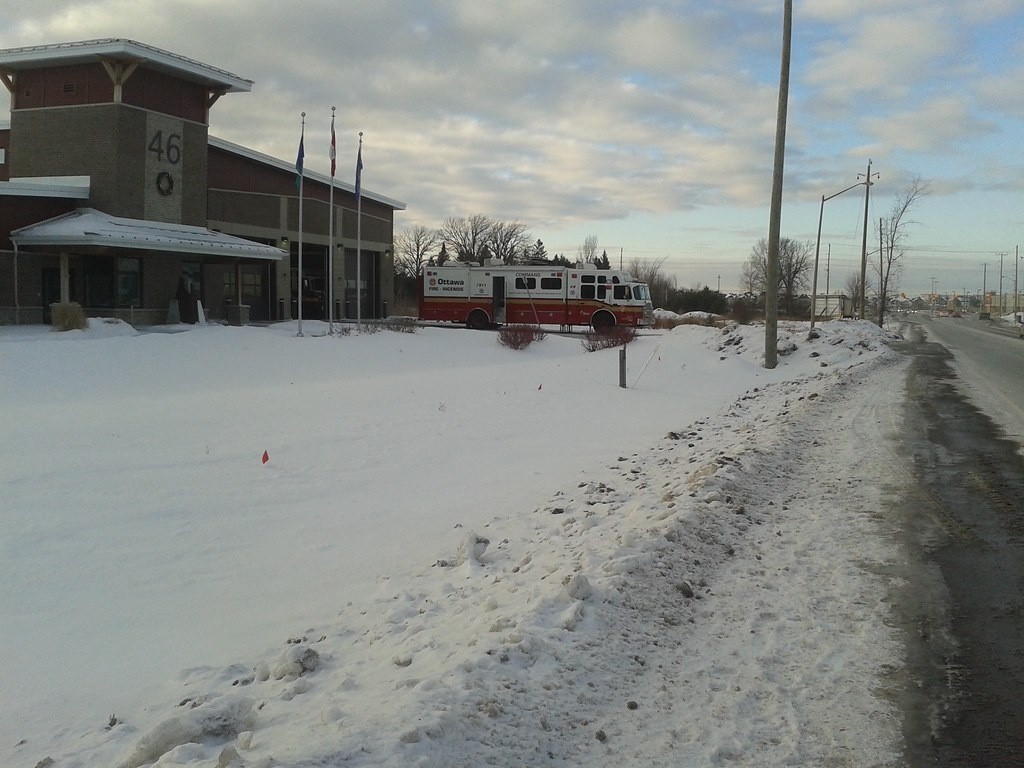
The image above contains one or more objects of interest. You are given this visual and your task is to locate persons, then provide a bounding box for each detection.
[175,271,198,324]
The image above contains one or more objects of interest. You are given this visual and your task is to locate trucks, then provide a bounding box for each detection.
[419,255,655,332]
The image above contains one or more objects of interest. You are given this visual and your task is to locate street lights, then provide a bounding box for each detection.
[810,180,874,330]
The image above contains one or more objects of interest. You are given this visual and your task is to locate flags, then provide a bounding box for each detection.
[328,121,337,177]
[354,149,363,197]
[294,136,305,188]
